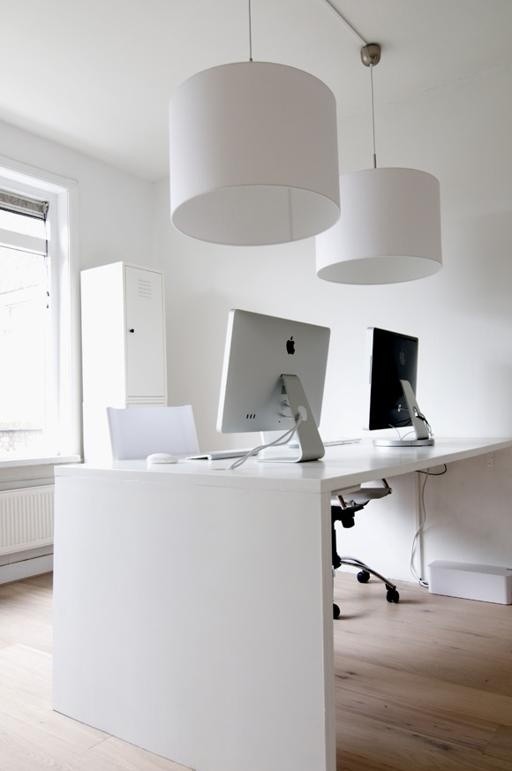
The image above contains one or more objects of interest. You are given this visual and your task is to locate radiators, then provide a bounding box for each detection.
[0,484,55,558]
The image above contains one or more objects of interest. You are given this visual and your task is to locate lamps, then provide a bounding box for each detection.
[169,0,341,246]
[315,42,442,285]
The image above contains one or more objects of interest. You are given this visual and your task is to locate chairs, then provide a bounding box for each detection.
[263,403,399,620]
[107,405,200,462]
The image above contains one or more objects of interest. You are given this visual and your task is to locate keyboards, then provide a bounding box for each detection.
[184,449,259,460]
[287,437,363,447]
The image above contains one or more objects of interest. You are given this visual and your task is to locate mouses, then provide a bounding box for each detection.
[146,453,177,464]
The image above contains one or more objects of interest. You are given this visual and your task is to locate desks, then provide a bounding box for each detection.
[50,434,512,771]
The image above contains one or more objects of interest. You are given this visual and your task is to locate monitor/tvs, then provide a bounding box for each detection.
[215,308,330,463]
[364,326,434,447]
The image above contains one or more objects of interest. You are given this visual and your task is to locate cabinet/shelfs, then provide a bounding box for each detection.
[80,261,168,463]
